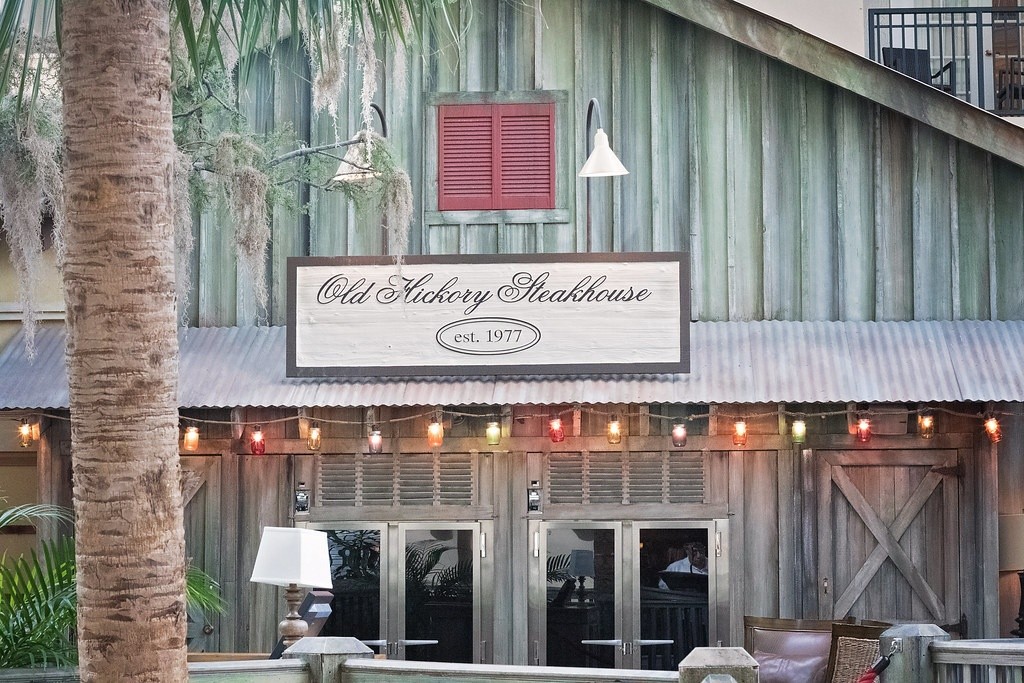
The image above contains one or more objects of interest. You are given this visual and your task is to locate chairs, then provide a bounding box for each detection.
[997,57,1024,111]
[882,47,956,98]
[742,615,950,683]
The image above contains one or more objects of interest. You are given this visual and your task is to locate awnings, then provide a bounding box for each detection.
[0,320,1024,407]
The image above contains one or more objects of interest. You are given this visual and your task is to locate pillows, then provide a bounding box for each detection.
[751,650,828,683]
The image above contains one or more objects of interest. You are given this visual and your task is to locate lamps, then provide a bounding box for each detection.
[250,526,334,647]
[333,103,390,256]
[578,98,629,252]
[999,514,1024,639]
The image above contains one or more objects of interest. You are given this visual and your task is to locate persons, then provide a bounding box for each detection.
[659,535,708,589]
